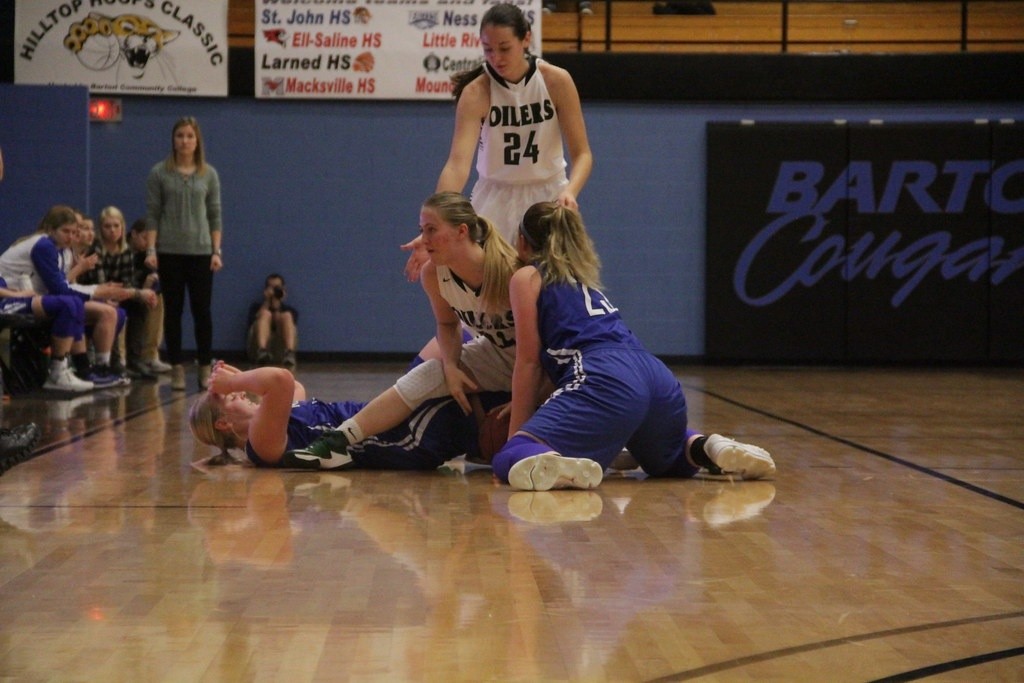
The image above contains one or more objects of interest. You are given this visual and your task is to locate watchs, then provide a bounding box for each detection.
[214,249,221,255]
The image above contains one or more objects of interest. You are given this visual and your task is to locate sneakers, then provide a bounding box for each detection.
[44,369,95,396]
[284,432,356,472]
[87,355,171,387]
[509,453,604,491]
[704,433,775,480]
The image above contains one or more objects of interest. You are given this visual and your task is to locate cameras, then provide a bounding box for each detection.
[274,287,284,299]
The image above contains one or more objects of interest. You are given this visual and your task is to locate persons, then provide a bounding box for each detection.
[0,206,171,401]
[188,320,512,470]
[491,202,777,492]
[285,192,640,471]
[245,274,298,372]
[144,117,222,390]
[400,3,592,283]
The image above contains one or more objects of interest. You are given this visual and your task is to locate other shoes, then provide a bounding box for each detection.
[0,422,36,452]
[199,368,211,390]
[171,367,187,389]
[284,350,296,364]
[260,350,271,363]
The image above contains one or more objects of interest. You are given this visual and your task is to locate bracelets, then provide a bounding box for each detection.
[135,288,139,298]
[145,247,155,254]
